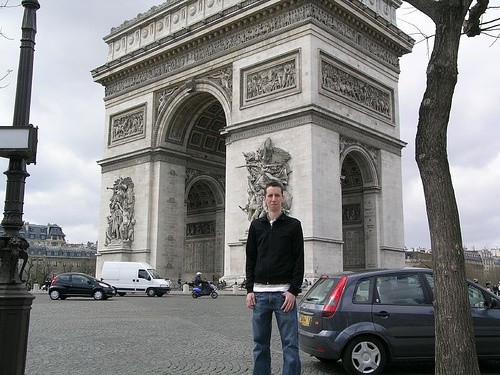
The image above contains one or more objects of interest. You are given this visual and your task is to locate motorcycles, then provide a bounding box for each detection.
[191,280,219,298]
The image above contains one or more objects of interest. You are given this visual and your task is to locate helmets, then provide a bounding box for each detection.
[197,272,202,275]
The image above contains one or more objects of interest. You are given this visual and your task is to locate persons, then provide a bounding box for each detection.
[245,182,304,375]
[473,277,500,308]
[193,272,209,293]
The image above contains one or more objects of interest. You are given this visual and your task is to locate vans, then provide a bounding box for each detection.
[102,262,171,297]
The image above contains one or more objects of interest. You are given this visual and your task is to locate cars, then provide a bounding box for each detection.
[49,272,116,302]
[298,267,500,375]
